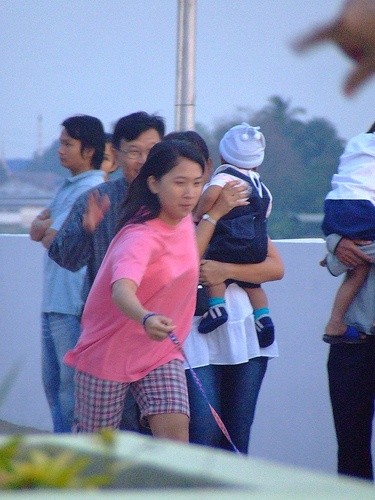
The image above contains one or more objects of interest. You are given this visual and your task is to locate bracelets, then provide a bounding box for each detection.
[142,313,158,328]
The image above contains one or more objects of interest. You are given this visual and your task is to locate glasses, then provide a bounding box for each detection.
[114,147,150,159]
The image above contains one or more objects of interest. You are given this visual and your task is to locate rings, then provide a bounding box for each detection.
[201,282,207,288]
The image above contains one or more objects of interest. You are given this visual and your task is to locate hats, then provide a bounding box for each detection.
[219,122,265,169]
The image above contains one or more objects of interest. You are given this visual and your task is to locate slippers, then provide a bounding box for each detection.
[323,325,369,344]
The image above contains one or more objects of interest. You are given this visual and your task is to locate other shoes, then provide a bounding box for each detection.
[198,304,228,334]
[255,314,275,349]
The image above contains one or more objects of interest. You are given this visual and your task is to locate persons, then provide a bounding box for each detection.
[62,139,204,443]
[160,131,279,456]
[320,219,374,481]
[47,111,168,318]
[192,121,275,348]
[320,123,375,346]
[29,114,106,434]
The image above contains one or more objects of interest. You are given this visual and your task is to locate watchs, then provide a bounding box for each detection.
[202,212,217,227]
